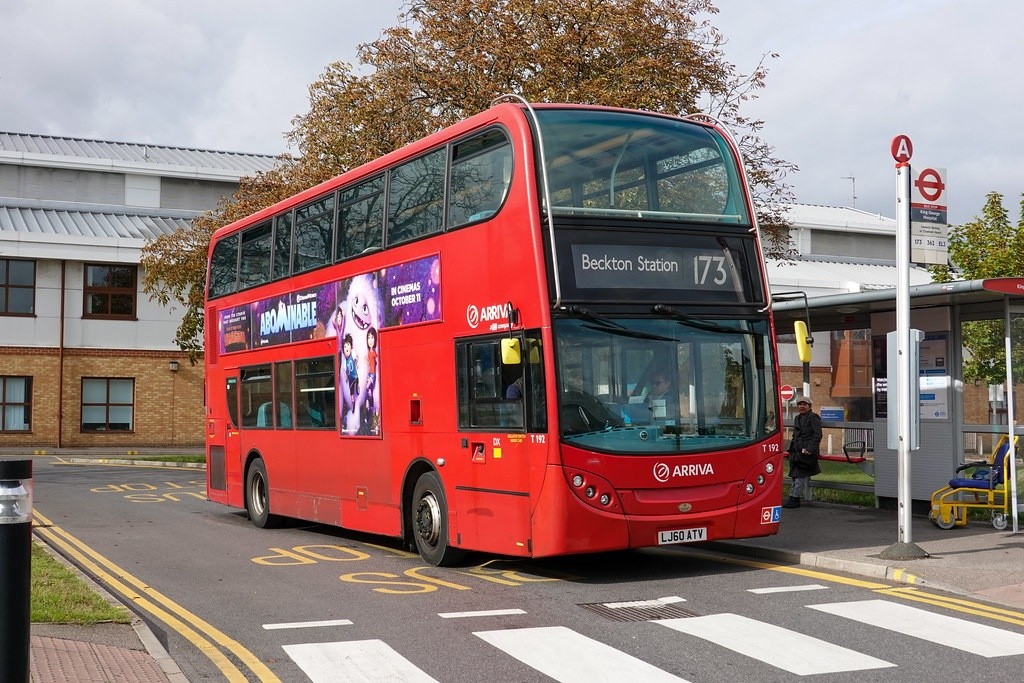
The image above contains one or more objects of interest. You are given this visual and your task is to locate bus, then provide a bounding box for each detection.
[202,92,815,568]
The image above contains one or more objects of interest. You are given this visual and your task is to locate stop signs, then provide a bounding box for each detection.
[780,384,795,400]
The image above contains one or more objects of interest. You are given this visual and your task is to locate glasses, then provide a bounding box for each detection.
[649,380,667,386]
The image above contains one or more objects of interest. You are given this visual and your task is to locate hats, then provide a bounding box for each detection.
[796,397,813,406]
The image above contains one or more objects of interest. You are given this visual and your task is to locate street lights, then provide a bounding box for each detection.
[841,176,857,209]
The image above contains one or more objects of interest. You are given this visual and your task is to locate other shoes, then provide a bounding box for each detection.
[783,496,800,508]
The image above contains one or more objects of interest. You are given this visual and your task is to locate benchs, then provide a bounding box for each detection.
[782,441,866,463]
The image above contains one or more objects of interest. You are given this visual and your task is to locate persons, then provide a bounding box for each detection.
[506,364,546,428]
[323,378,334,426]
[644,371,674,420]
[782,396,823,508]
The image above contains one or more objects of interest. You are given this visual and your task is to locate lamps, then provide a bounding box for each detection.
[170,362,179,372]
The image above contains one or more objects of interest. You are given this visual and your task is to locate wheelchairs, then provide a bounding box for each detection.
[929,435,1020,531]
